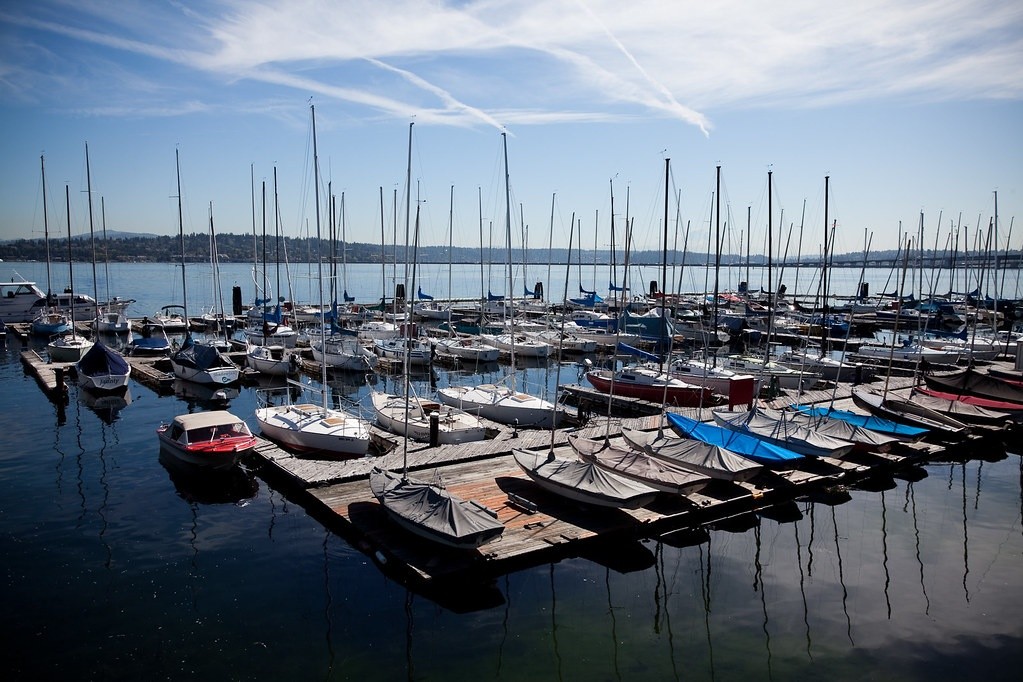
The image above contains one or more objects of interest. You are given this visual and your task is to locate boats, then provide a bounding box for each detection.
[155,411,257,476]
[154,305,192,334]
[121,316,172,359]
[0,267,137,323]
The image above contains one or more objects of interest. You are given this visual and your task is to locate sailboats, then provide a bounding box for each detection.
[71,141,132,394]
[252,95,377,455]
[165,149,242,385]
[49,182,94,363]
[365,207,505,550]
[193,121,1023,497]
[508,193,662,512]
[88,194,134,358]
[32,151,76,338]
